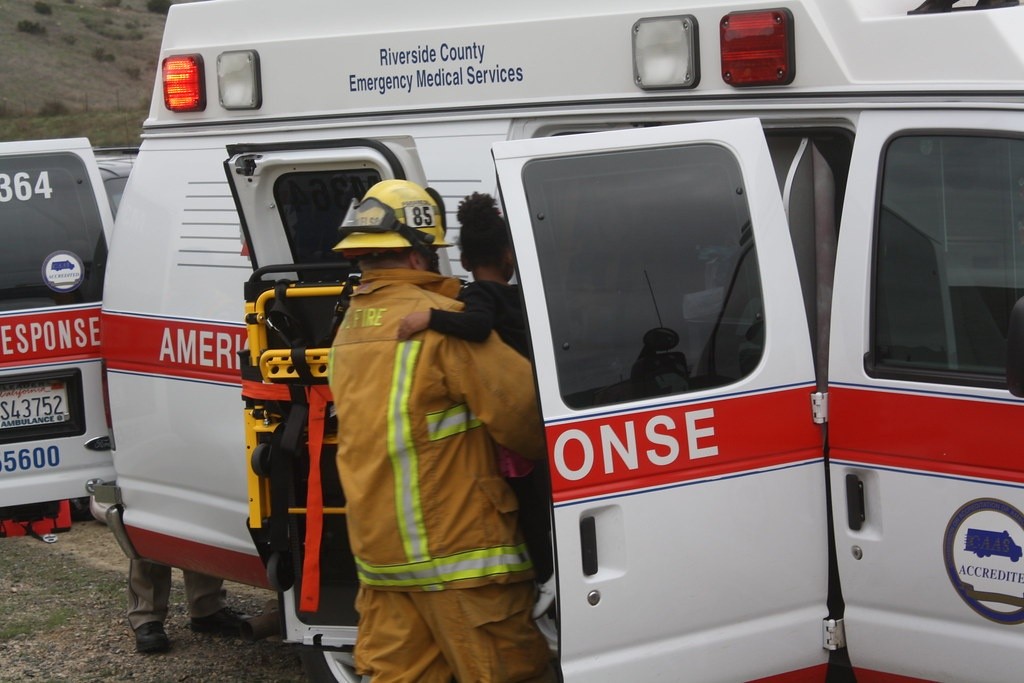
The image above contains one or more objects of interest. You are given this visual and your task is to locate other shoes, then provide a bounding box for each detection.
[532,572,556,620]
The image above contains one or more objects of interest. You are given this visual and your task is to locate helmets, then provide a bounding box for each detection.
[332,179,454,251]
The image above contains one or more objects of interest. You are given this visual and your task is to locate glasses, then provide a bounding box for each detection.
[338,198,435,261]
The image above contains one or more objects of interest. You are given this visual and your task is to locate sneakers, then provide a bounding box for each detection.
[135,622,168,651]
[192,607,247,635]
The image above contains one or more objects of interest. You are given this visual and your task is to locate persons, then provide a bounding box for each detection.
[127,559,256,651]
[328,179,560,682]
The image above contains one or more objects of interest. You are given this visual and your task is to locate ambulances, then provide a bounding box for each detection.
[0,1,1024,683]
